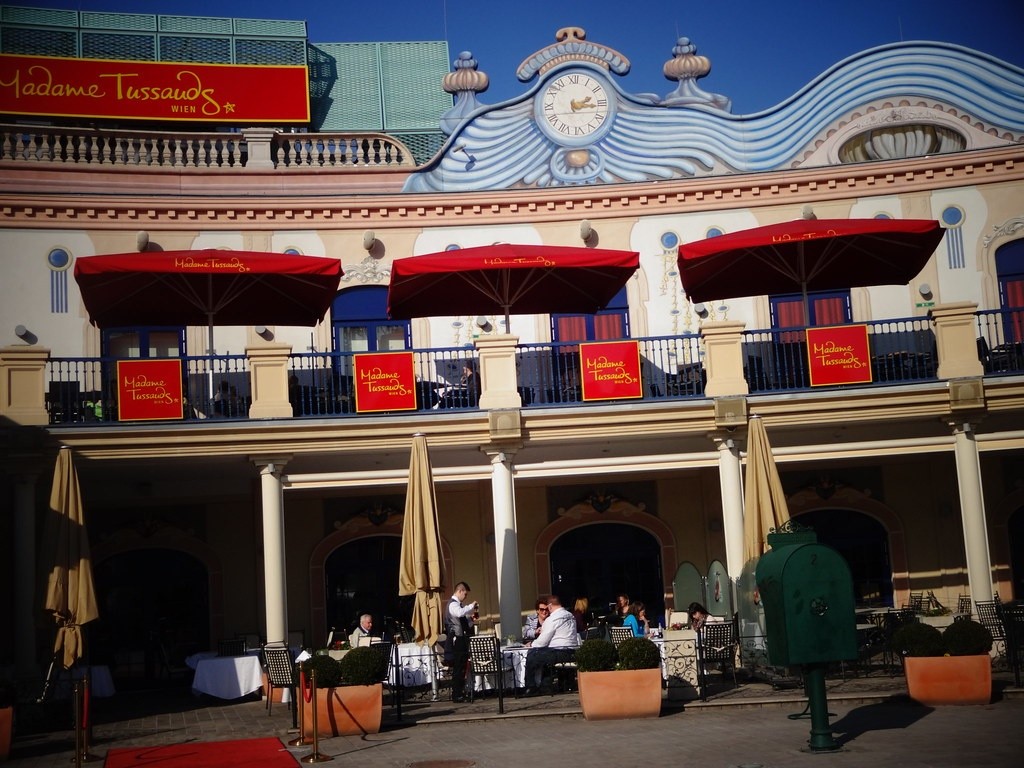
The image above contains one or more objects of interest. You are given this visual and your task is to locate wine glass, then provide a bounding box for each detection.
[652,630,655,636]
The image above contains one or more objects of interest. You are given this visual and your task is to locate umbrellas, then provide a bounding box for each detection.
[74,249,344,416]
[742,415,793,599]
[398,434,449,701]
[32,446,101,768]
[386,244,640,334]
[677,219,947,326]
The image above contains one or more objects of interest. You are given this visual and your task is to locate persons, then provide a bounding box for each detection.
[444,582,479,703]
[573,597,589,640]
[439,360,481,408]
[350,614,372,648]
[623,600,650,638]
[520,595,578,696]
[87,390,102,417]
[523,598,551,638]
[615,593,629,616]
[687,602,717,676]
[214,381,228,401]
[288,376,302,417]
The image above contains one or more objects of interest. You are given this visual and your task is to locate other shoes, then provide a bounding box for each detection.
[519,686,538,698]
[453,698,472,703]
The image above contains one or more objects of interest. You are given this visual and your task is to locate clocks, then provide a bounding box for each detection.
[535,68,617,145]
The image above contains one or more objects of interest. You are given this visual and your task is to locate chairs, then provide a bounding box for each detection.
[469,634,517,699]
[551,626,600,697]
[260,642,298,716]
[701,621,737,686]
[607,623,635,649]
[854,588,1024,676]
[976,336,1011,375]
[667,608,727,629]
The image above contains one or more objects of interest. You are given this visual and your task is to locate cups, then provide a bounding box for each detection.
[316,649,329,656]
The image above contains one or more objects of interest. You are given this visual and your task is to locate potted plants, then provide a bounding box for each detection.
[296,645,390,739]
[577,636,662,719]
[903,619,993,703]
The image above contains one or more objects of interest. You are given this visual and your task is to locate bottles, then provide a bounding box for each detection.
[658,623,663,638]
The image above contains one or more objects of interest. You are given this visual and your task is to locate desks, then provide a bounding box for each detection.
[649,628,699,690]
[184,647,263,699]
[854,608,905,678]
[871,351,930,381]
[52,666,116,698]
[383,642,444,687]
[474,644,533,691]
[995,342,1024,373]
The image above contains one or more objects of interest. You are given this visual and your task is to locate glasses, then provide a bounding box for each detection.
[539,607,550,612]
